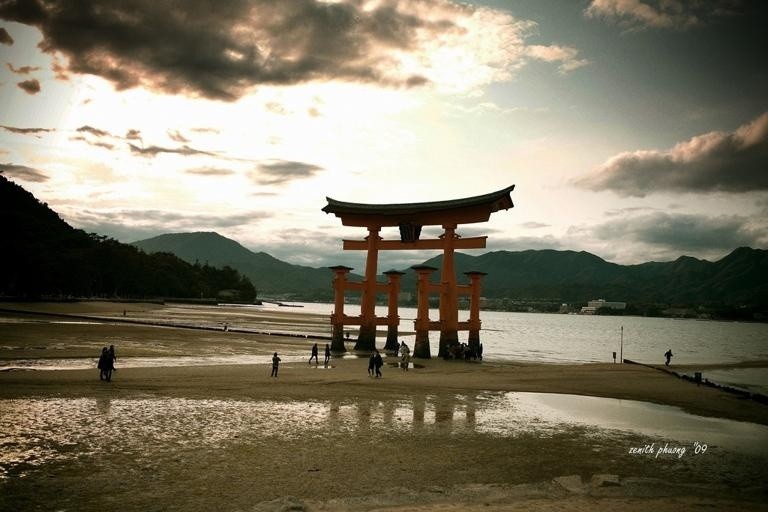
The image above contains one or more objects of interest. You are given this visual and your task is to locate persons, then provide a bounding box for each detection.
[270,353,281,377]
[374,350,383,378]
[309,343,318,365]
[325,344,330,364]
[393,340,410,371]
[106,345,116,382]
[98,347,111,382]
[665,349,673,366]
[443,341,483,362]
[368,353,375,376]
